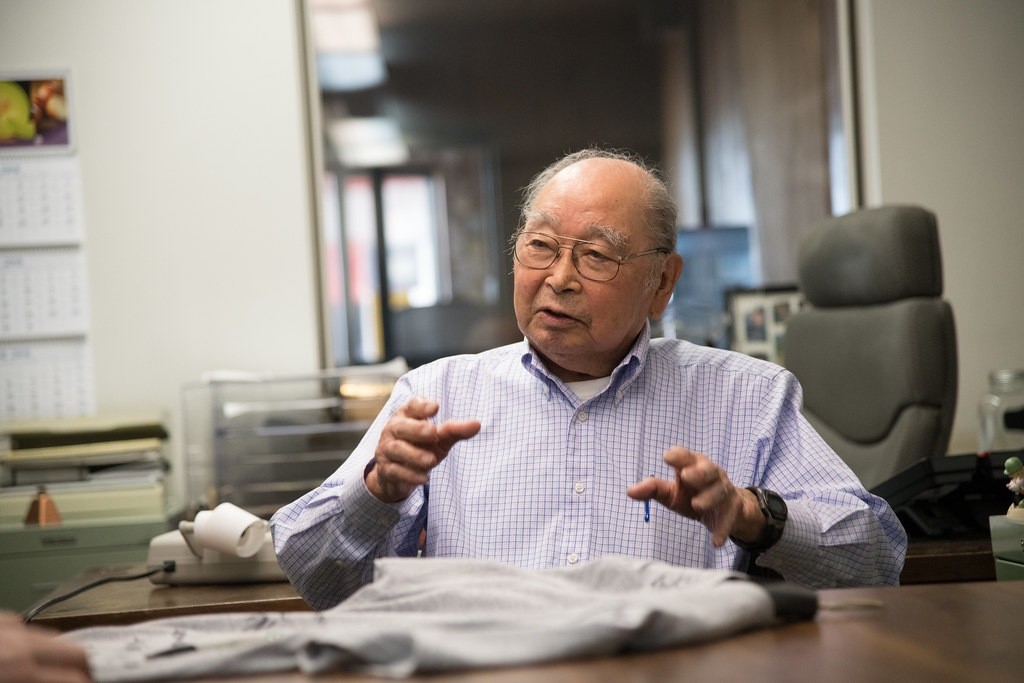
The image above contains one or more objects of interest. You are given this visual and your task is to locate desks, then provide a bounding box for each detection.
[23,541,1024,683]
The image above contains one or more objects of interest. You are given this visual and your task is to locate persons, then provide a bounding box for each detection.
[0,608,96,683]
[269,142,909,614]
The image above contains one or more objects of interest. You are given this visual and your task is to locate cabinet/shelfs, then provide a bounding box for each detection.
[188,359,414,518]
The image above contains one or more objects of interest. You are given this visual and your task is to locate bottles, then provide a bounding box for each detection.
[978,367,1024,452]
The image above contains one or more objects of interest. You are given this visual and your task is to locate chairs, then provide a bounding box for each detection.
[784,205,958,492]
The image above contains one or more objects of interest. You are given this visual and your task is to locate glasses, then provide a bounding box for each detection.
[514,224,670,282]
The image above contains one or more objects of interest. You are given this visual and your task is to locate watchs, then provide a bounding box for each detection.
[727,485,788,554]
